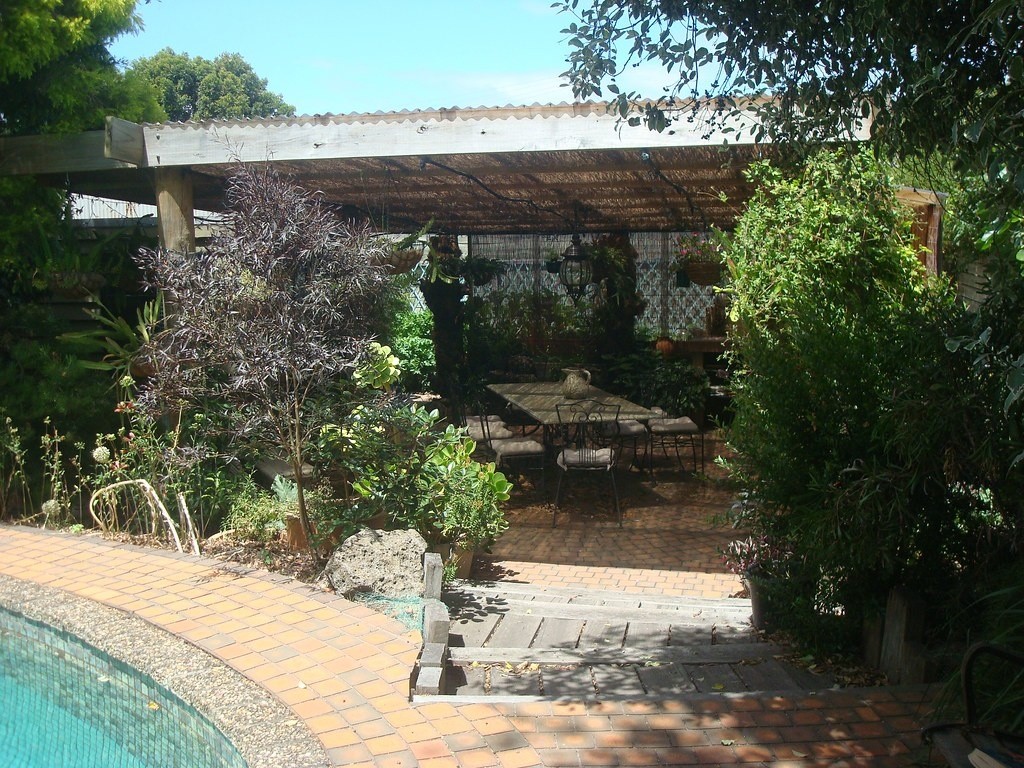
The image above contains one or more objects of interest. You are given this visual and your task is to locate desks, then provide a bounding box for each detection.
[486,381,661,490]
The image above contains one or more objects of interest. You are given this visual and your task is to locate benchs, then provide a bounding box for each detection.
[921,641,1024,768]
[247,440,321,487]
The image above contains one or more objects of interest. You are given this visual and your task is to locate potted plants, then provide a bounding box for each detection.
[545,252,562,273]
[442,255,510,287]
[283,340,513,579]
[508,344,599,380]
[589,245,640,306]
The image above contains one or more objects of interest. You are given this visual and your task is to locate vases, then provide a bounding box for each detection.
[684,261,726,285]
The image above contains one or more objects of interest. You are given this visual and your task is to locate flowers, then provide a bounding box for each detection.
[672,230,728,264]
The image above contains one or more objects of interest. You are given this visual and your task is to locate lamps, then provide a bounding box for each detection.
[557,208,592,307]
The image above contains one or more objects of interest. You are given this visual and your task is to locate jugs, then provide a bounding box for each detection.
[561,367,591,399]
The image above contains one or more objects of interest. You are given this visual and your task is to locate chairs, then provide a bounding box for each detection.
[550,400,624,528]
[609,382,707,480]
[448,378,548,499]
